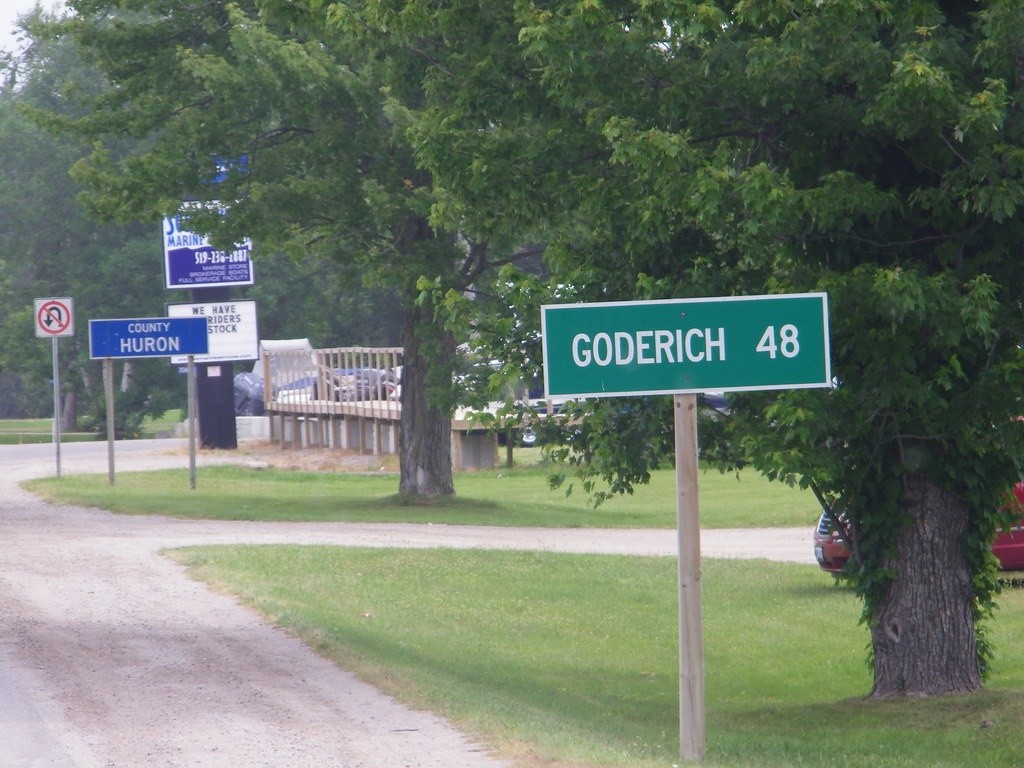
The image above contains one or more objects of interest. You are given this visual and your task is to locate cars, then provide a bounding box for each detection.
[810,413,1024,580]
[231,354,737,448]
